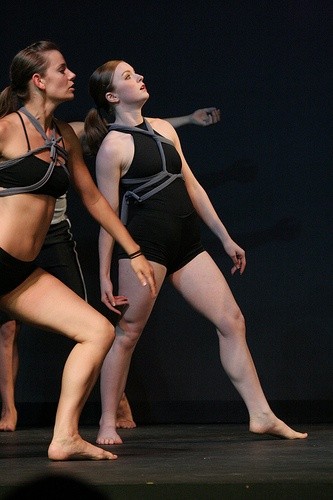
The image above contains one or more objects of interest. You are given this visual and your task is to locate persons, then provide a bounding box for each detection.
[82,60,308,440]
[0,41,159,461]
[0,107,221,432]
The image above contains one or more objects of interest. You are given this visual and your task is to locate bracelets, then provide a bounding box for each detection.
[128,248,144,260]
